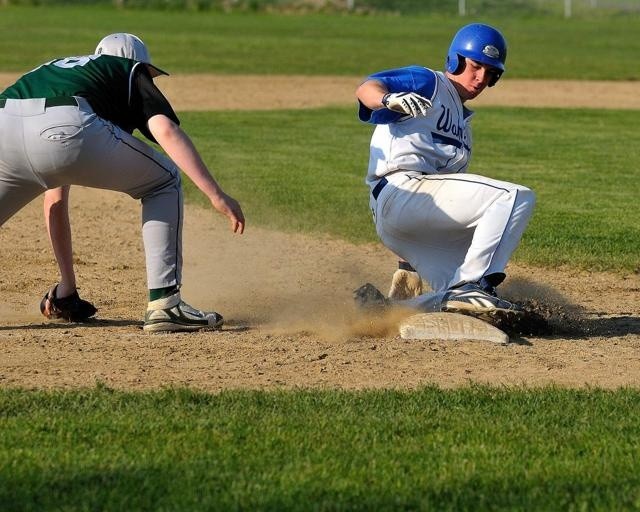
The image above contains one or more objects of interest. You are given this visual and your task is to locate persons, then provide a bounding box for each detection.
[0,31,245,334]
[352,23,537,339]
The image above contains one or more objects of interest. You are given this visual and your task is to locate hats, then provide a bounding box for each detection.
[94,33,171,78]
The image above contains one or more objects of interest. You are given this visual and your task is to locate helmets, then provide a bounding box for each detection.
[445,23,506,88]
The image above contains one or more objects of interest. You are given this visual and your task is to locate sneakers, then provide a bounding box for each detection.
[354,283,389,316]
[143,300,225,331]
[441,270,527,325]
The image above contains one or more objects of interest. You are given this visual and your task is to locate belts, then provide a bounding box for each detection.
[0,97,78,108]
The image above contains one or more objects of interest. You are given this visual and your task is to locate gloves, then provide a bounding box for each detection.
[382,91,434,119]
[388,261,423,301]
